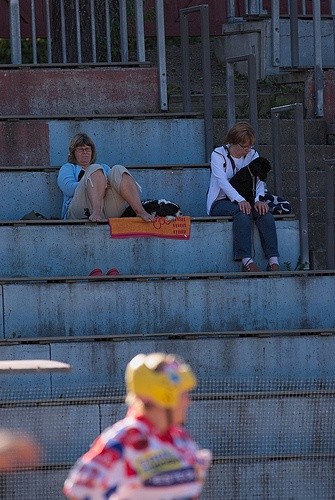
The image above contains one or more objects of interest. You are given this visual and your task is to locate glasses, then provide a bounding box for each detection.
[238,143,255,152]
[77,148,91,151]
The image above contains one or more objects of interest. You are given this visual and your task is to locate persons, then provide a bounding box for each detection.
[57,132,157,223]
[206,121,281,278]
[62,351,213,500]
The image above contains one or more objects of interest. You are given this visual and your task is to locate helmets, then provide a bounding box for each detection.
[125,352,196,405]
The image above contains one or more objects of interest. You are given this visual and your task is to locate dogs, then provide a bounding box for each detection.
[226,157,272,208]
[120,199,183,221]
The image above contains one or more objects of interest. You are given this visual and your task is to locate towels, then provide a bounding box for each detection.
[107,216,192,240]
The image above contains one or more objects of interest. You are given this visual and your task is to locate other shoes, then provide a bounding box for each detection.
[267,262,280,271]
[242,261,257,271]
[106,267,119,275]
[89,268,102,276]
